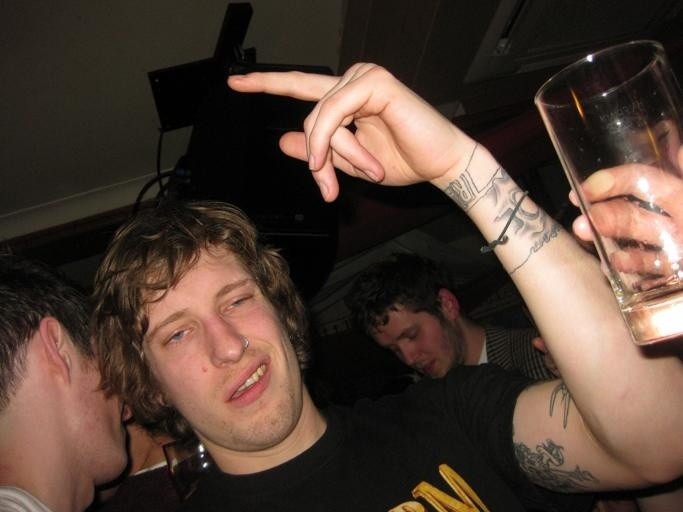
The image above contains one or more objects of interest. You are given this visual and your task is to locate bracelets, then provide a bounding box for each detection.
[479,190,530,254]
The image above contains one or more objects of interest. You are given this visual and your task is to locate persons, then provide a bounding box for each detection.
[97,60,682,512]
[2,248,132,511]
[348,250,575,382]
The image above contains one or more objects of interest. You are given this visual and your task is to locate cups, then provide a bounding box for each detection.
[533,41,683,346]
